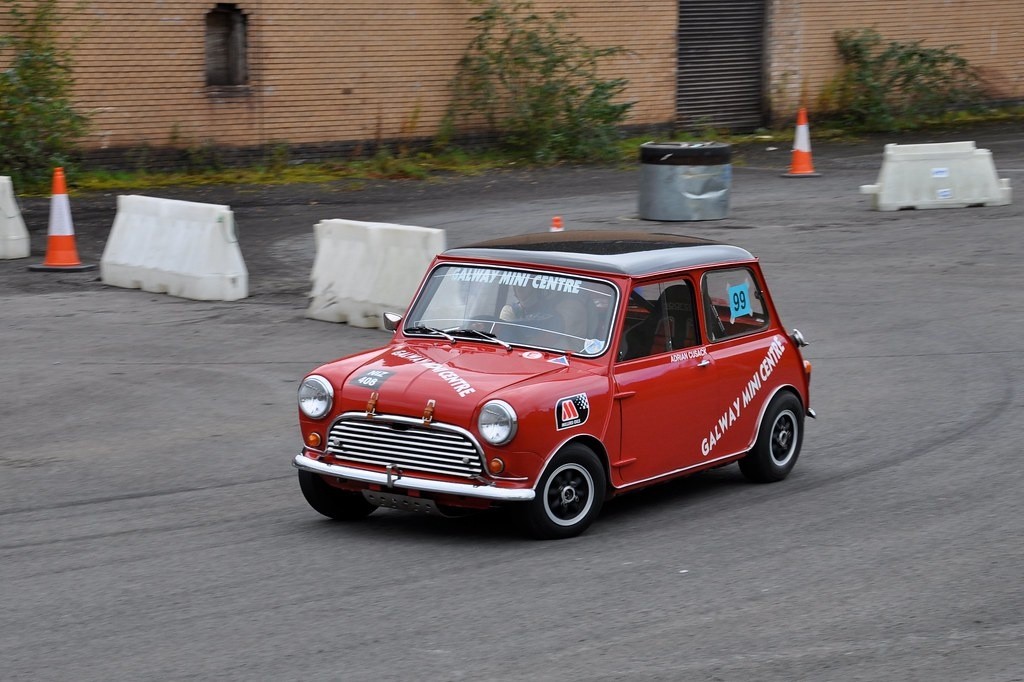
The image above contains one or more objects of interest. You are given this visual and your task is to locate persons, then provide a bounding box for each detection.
[465,276,567,345]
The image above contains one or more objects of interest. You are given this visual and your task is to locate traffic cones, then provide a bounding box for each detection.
[781,106,825,179]
[28,167,99,274]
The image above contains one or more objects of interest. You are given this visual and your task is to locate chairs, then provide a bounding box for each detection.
[629,284,712,360]
[548,292,599,352]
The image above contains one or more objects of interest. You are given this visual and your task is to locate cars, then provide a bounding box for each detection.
[291,229,818,539]
